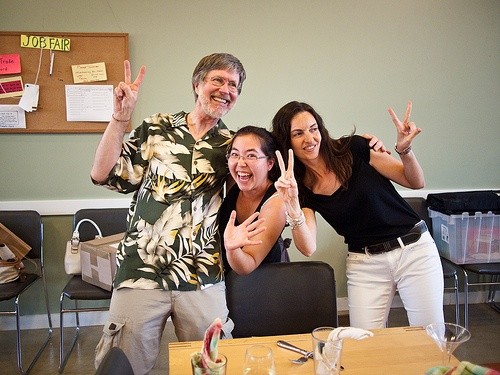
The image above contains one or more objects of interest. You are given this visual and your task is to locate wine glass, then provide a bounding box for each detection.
[426,322,472,366]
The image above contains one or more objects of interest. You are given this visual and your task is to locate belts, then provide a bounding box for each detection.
[348,223,428,254]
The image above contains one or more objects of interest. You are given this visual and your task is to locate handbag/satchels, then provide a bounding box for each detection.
[0,257,28,284]
[64,219,102,274]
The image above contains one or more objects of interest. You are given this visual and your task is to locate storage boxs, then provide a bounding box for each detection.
[80,232,126,292]
[427,206,500,265]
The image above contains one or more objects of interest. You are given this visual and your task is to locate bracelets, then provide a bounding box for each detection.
[112,114,130,122]
[394,146,412,155]
[287,211,304,230]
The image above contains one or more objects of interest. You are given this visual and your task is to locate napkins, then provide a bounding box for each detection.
[318,327,373,375]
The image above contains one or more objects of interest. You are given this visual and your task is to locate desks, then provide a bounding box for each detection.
[168,326,461,375]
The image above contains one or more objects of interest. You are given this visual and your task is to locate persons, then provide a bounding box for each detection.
[216,126,293,275]
[272,101,446,337]
[89,52,391,375]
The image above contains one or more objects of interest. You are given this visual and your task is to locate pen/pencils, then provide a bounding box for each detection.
[50,52,55,75]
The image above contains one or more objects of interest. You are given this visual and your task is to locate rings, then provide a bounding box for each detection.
[407,122,410,127]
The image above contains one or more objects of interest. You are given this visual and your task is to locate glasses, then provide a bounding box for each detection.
[204,77,239,93]
[225,154,269,163]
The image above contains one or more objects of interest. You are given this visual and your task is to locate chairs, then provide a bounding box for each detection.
[58,207,128,372]
[401,197,500,336]
[0,210,53,375]
[225,261,338,339]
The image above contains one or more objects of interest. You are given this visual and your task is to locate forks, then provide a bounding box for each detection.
[289,350,313,363]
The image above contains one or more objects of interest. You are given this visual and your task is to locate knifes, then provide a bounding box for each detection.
[277,339,344,370]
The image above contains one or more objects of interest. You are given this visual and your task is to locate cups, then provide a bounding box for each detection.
[191,351,228,375]
[242,345,277,375]
[311,326,343,375]
